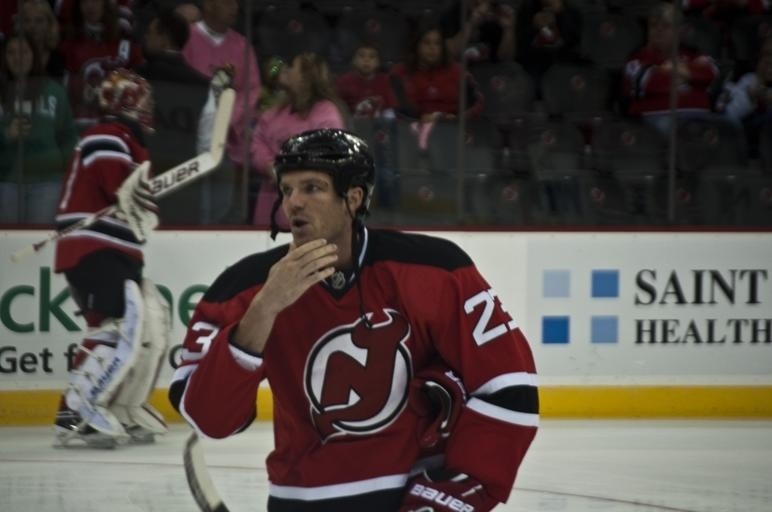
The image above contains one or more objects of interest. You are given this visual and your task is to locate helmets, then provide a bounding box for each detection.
[94,67,158,144]
[272,127,376,231]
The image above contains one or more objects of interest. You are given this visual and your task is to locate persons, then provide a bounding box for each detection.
[165,126,539,512]
[1,0,771,228]
[52,60,175,443]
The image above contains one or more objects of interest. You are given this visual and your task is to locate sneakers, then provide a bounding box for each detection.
[56,402,94,433]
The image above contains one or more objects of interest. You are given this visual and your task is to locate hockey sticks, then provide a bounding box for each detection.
[11,88,236,262]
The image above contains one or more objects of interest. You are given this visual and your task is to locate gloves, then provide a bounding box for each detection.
[398,468,499,512]
[411,359,471,452]
